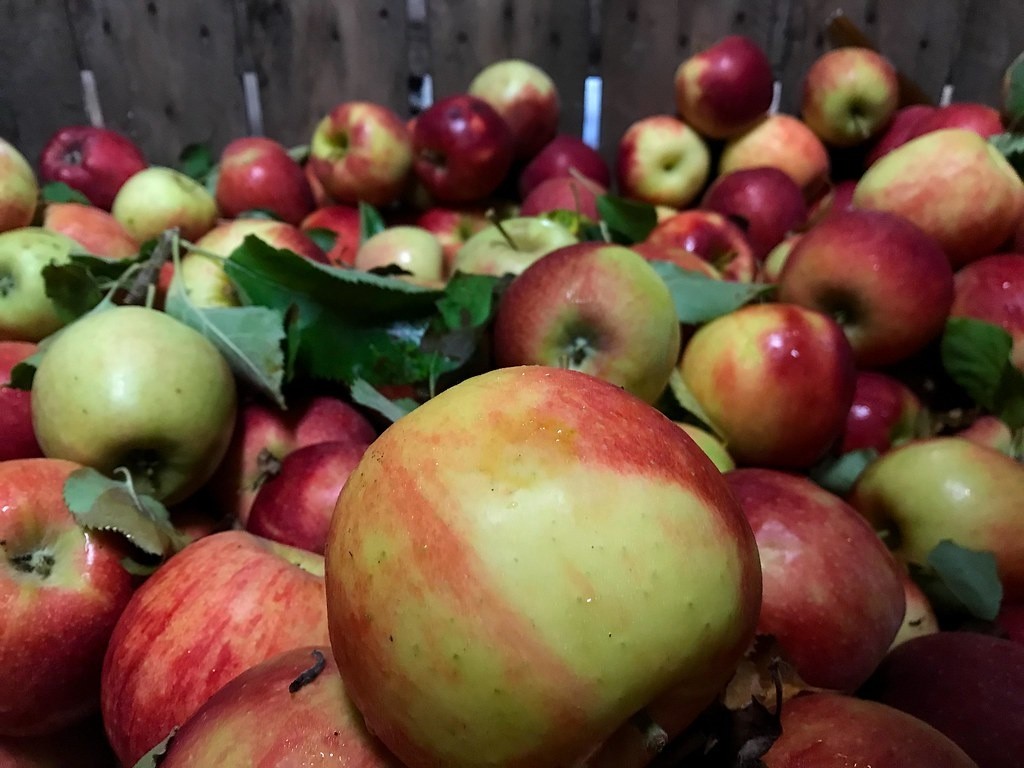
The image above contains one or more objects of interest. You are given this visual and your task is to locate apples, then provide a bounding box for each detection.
[0,35,1024,768]
